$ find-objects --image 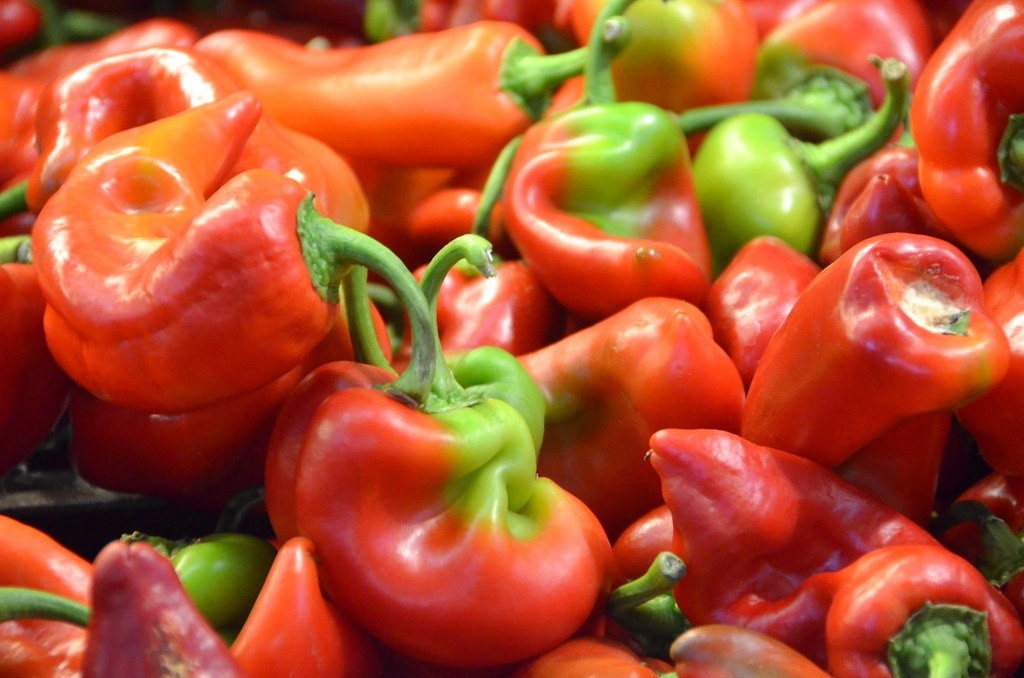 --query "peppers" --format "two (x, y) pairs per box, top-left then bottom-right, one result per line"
(0, 0), (1024, 678)
